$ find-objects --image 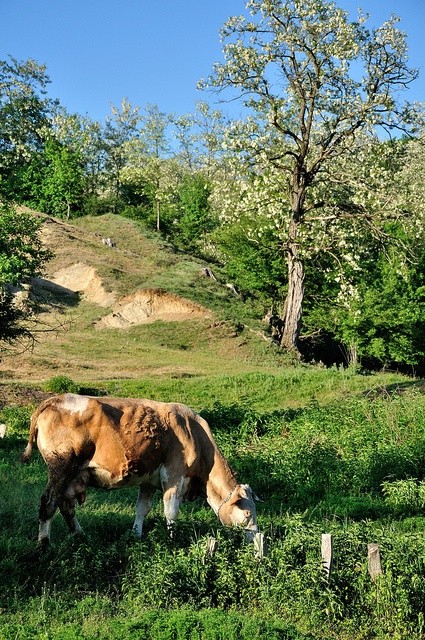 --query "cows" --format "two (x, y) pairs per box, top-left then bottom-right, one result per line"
(21, 394), (265, 551)
